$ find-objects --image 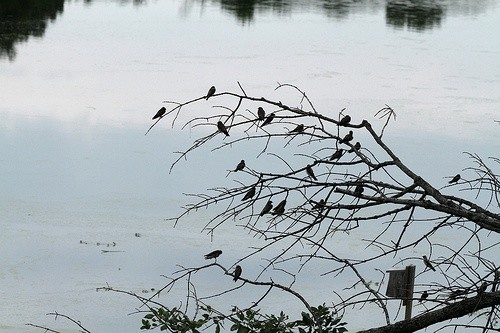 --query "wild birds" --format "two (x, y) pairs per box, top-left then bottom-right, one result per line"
(206, 85), (216, 100)
(419, 290), (429, 303)
(243, 186), (257, 201)
(288, 123), (304, 134)
(260, 199), (289, 217)
(330, 149), (344, 162)
(259, 113), (276, 129)
(234, 159), (246, 173)
(307, 165), (318, 181)
(311, 198), (326, 210)
(217, 121), (230, 137)
(354, 184), (365, 194)
(258, 107), (266, 122)
(204, 248), (223, 260)
(336, 115), (351, 127)
(152, 106), (166, 119)
(348, 142), (362, 153)
(340, 131), (353, 144)
(449, 174), (462, 184)
(234, 265), (243, 280)
(421, 256), (437, 272)
